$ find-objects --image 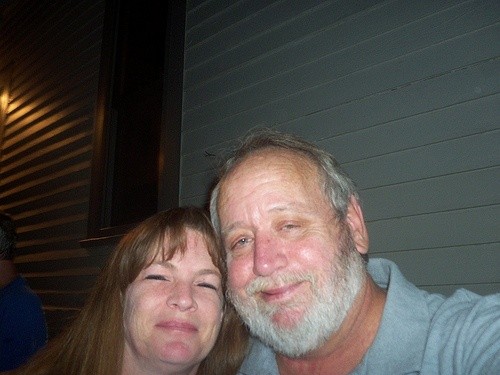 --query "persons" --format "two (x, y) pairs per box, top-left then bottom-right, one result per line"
(0, 206), (251, 375)
(0, 216), (48, 375)
(209, 128), (500, 375)
